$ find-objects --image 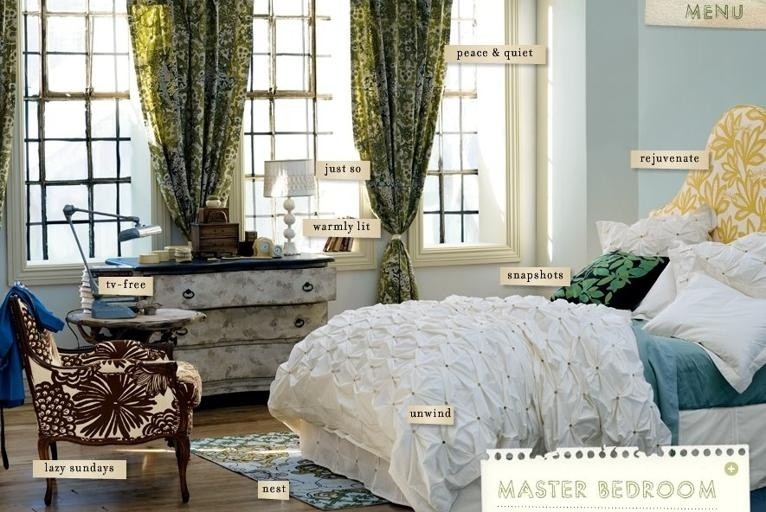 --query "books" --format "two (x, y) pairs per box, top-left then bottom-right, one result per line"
(79, 266), (144, 315)
(316, 215), (356, 252)
